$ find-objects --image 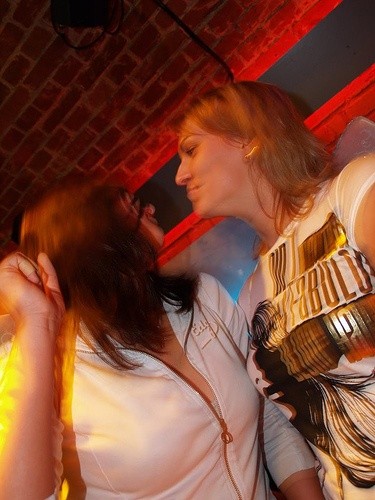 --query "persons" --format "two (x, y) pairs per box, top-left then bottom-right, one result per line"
(170, 81), (375, 500)
(2, 178), (330, 500)
